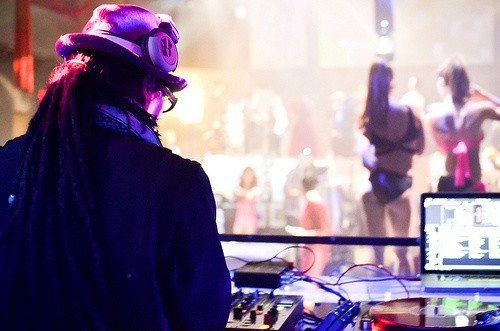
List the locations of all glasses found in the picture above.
[152,76,179,113]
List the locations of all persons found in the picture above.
[351,62,425,277]
[0,4,232,331]
[428,64,500,193]
[283,145,331,236]
[222,166,262,234]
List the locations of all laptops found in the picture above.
[420,192,500,291]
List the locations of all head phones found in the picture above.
[143,14,180,74]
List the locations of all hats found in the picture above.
[52,3,187,93]
